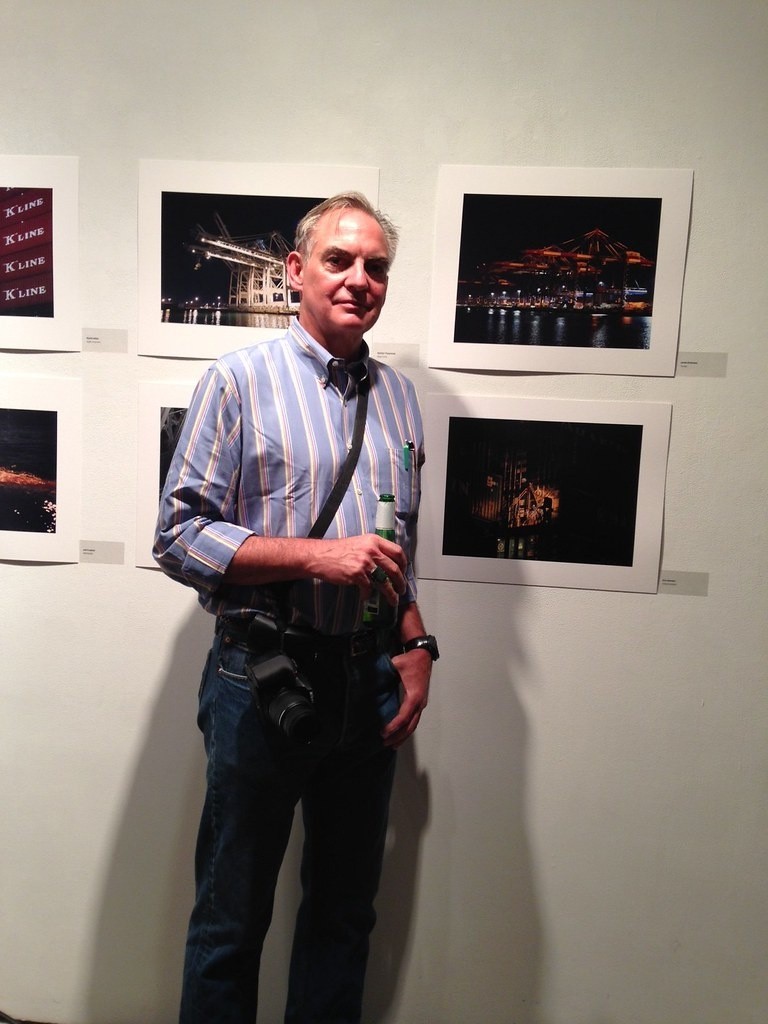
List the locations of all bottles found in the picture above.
[363,495,398,629]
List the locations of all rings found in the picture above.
[369,566,389,584]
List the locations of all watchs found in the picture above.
[402,634,440,662]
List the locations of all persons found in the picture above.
[150,192,432,1024]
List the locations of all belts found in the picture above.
[214,615,396,658]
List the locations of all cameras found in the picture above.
[244,653,320,745]
